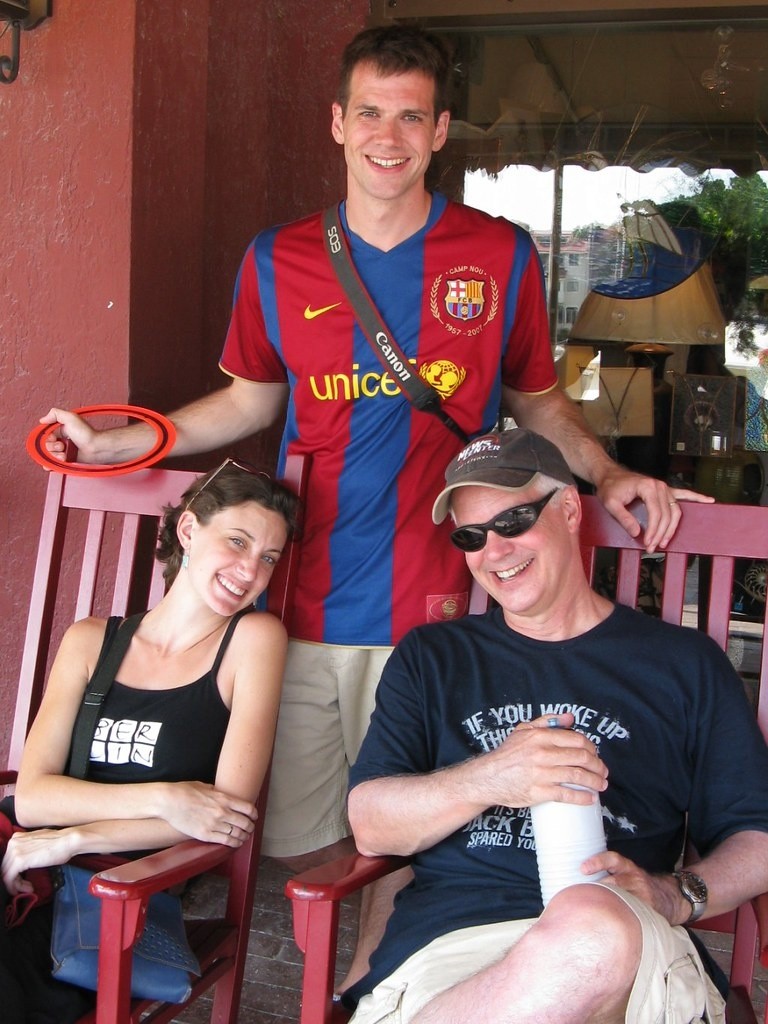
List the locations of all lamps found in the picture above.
[568,261,730,486]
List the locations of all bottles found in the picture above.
[529,716,609,908]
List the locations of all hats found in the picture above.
[432,428,577,525]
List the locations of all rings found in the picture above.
[227,824,233,836]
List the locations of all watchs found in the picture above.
[672,871,707,926]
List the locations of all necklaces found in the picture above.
[599,367,640,462]
[679,376,728,456]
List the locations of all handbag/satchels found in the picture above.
[52,865,202,1004]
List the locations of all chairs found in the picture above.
[285,494,768,1023]
[1,438,311,1024]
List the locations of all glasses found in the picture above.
[449,486,558,553]
[184,458,276,512]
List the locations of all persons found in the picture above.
[340,427,767,1024]
[39,23,716,1001]
[0,457,305,1024]
